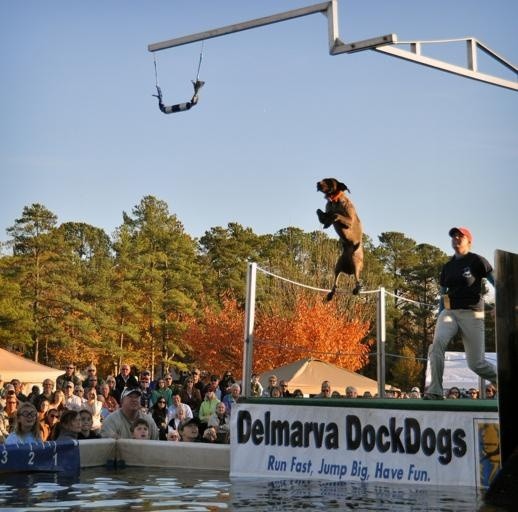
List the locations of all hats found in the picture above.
[4,383,15,394]
[120,386,143,399]
[448,227,473,240]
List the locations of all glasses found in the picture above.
[19,410,37,417]
[65,366,232,404]
[50,414,60,419]
[253,378,494,399]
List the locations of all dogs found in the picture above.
[316,178,364,301]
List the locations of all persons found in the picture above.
[422,227,497,400]
